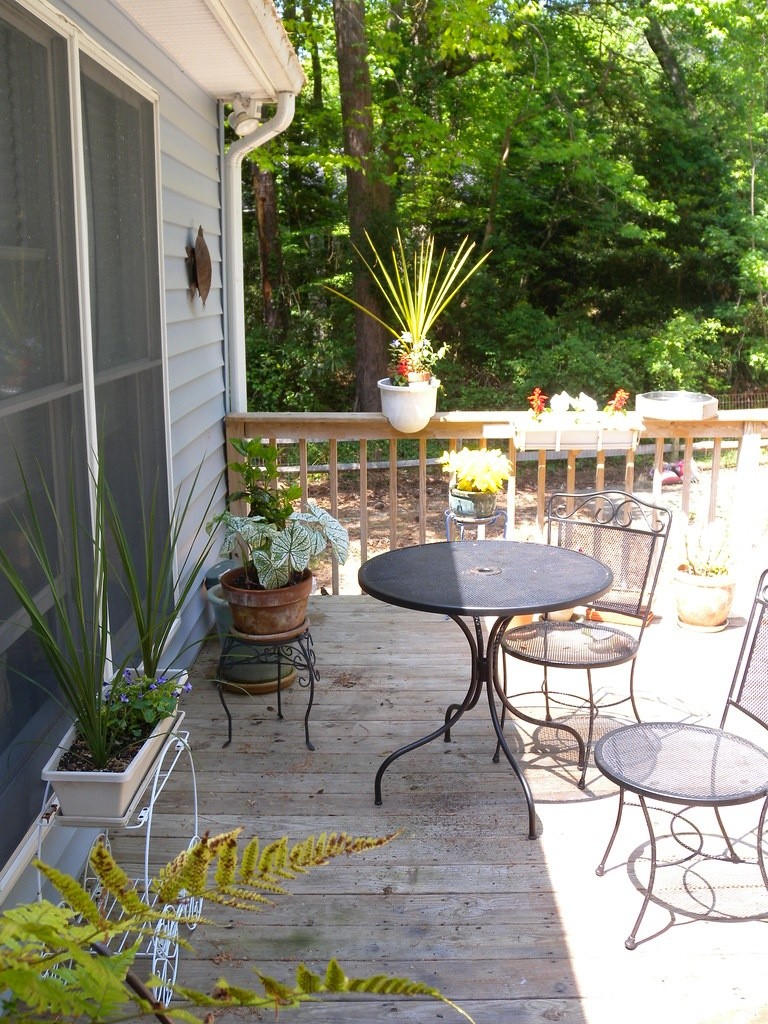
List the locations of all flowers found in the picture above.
[438,446,514,492]
[515,387,648,432]
[101,666,193,739]
[387,331,451,387]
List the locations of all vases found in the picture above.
[448,487,499,517]
[511,416,642,453]
[377,377,441,434]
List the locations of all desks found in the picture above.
[358,540,615,841]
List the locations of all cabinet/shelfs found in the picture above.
[35,731,205,1007]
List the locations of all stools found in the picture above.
[216,616,321,750]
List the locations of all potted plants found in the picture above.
[316,226,495,386]
[672,502,740,626]
[0,402,234,818]
[205,433,302,652]
[205,498,351,632]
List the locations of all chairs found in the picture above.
[491,489,671,790]
[594,570,768,951]
